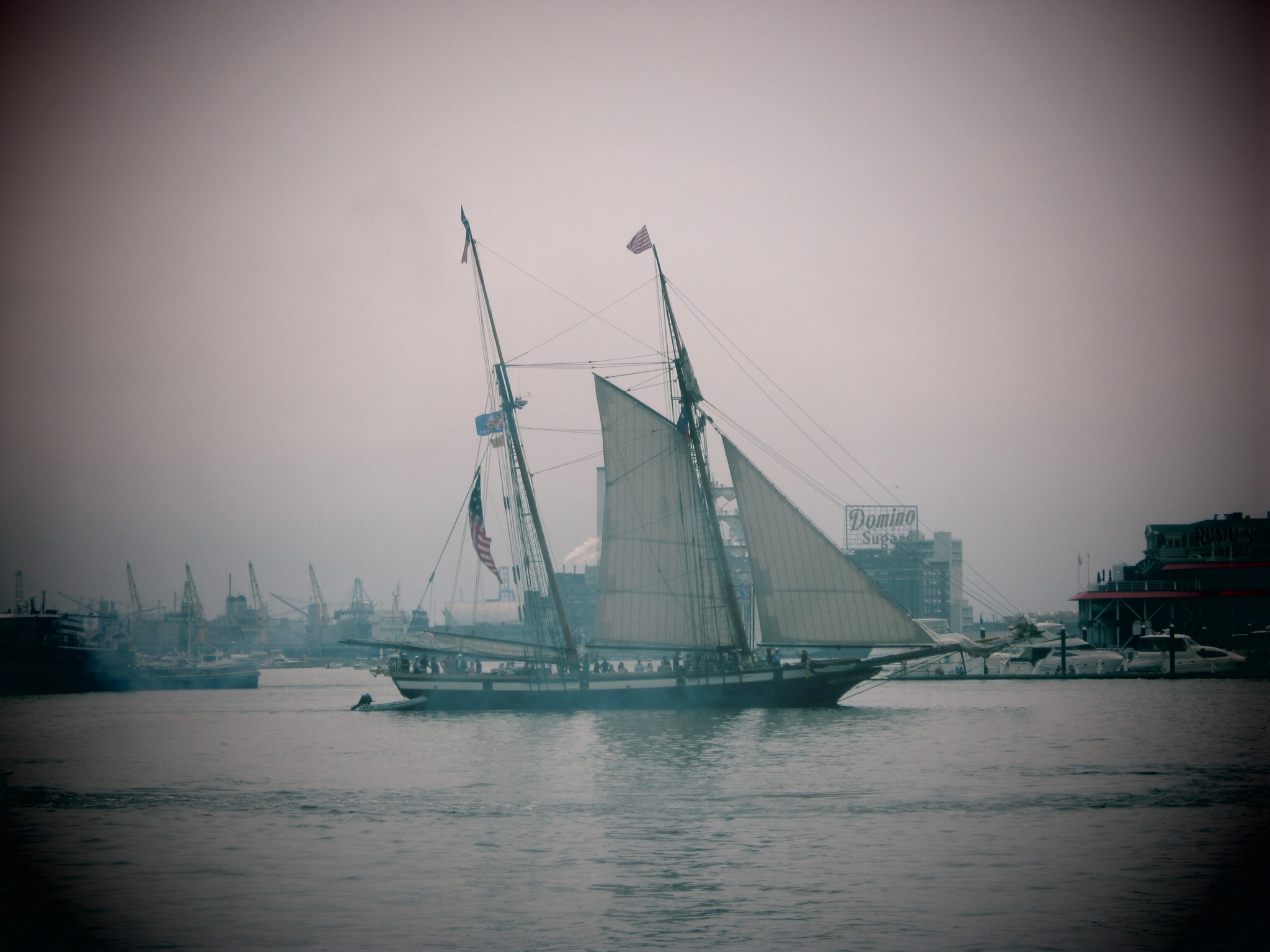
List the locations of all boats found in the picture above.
[260,648,305,668]
[0,573,264,691]
[353,656,372,670]
[359,695,426,712]
[325,660,344,669]
[864,617,1249,680]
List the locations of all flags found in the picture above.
[1079,555,1082,564]
[469,472,501,584]
[461,207,470,263]
[627,226,652,254]
[490,434,505,448]
[475,410,503,436]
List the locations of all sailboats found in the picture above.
[347,201,1040,713]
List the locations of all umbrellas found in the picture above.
[1109,569,1112,581]
[1102,569,1105,581]
[1097,572,1102,584]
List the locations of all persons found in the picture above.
[420,654,431,673]
[440,652,482,674]
[634,659,644,672]
[412,656,422,672]
[497,661,529,675]
[955,666,964,675]
[431,657,440,673]
[400,653,411,673]
[644,662,653,672]
[798,650,808,662]
[579,654,628,673]
[661,651,727,671]
[764,648,782,667]
